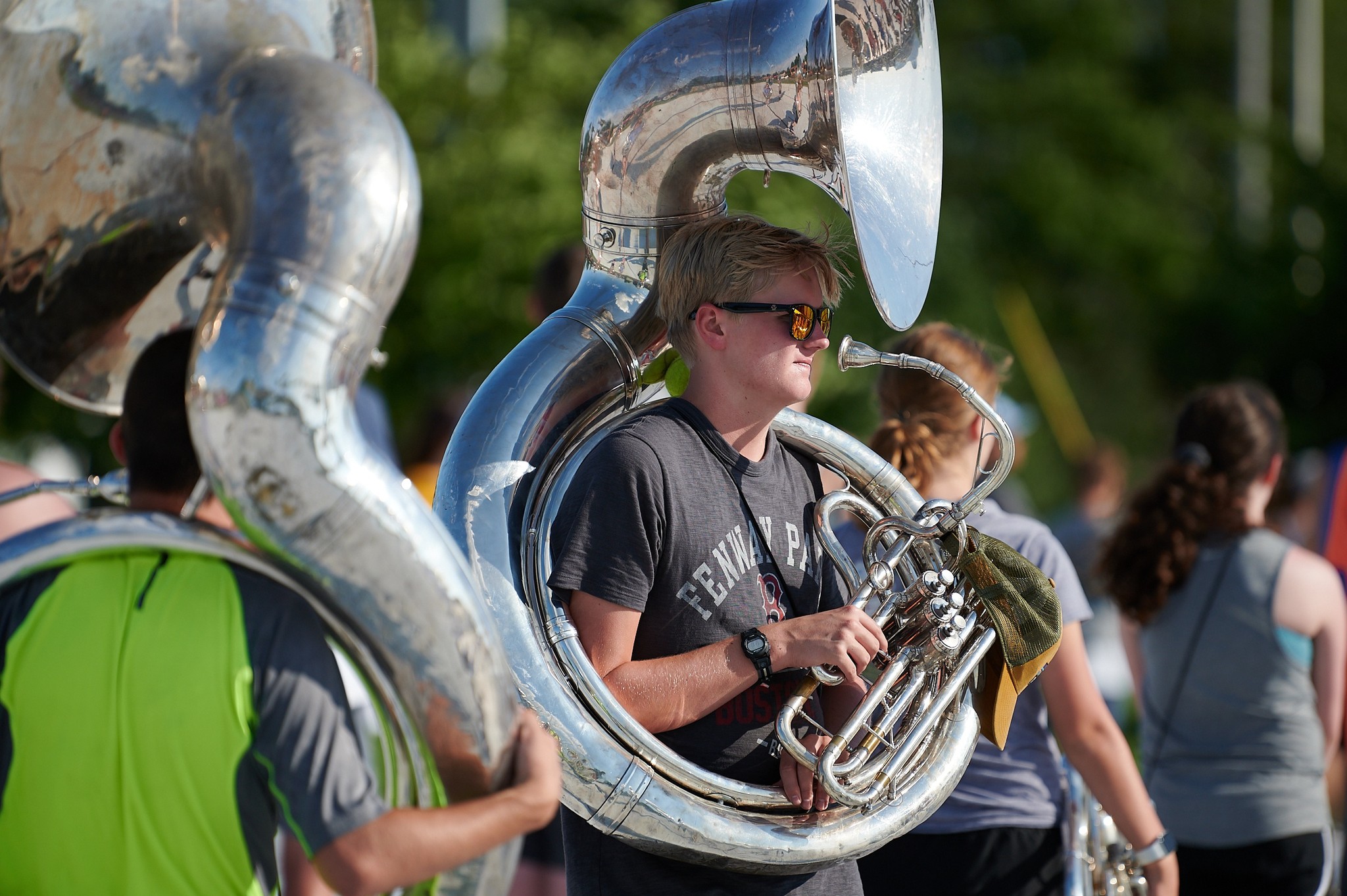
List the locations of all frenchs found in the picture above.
[431,0,1014,876]
[0,0,525,896]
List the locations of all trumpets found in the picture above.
[1061,753,1156,896]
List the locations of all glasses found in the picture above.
[685,300,833,342]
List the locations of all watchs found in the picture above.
[742,627,773,685]
[1136,832,1175,867]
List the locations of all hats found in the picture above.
[944,522,1062,751]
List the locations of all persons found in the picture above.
[973,397,1137,856]
[0,325,564,896]
[1099,378,1347,896]
[555,210,890,896]
[816,321,1183,896]
